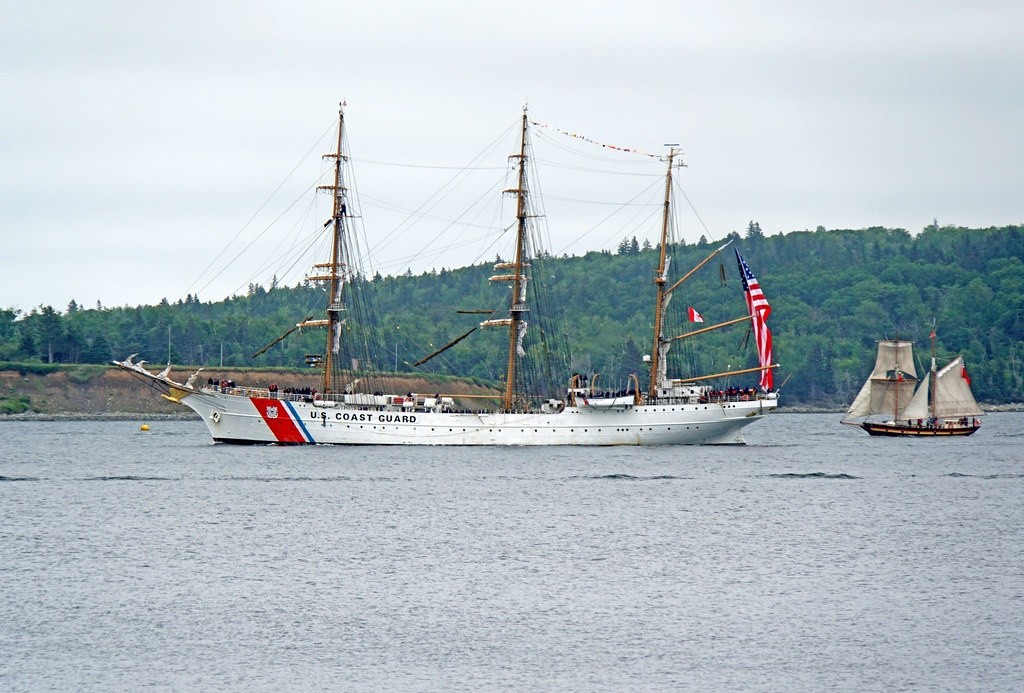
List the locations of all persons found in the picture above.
[926,416,939,429]
[374,390,384,397]
[958,416,969,427]
[767,385,775,394]
[434,392,440,398]
[565,389,643,407]
[918,419,923,428]
[221,379,235,394]
[269,383,278,398]
[972,415,975,427]
[208,377,219,391]
[699,386,758,404]
[282,386,359,401]
[908,419,912,426]
[407,391,414,401]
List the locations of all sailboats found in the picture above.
[112,100,781,449]
[840,315,986,437]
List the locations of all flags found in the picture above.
[688,307,704,323]
[733,243,774,394]
